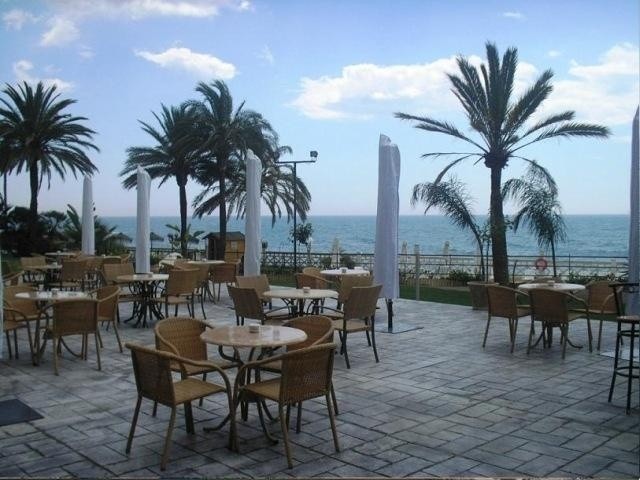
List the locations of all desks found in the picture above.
[200,323,306,449]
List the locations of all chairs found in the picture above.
[479,271,627,359]
[153,317,246,417]
[609,285,639,415]
[122,339,234,471]
[232,341,343,471]
[254,314,339,430]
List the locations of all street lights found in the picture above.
[271,150,319,278]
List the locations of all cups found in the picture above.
[303,287,310,293]
[547,281,555,286]
[248,324,259,333]
[341,269,346,273]
[202,259,206,262]
[51,288,58,295]
[147,272,153,278]
[52,262,57,266]
